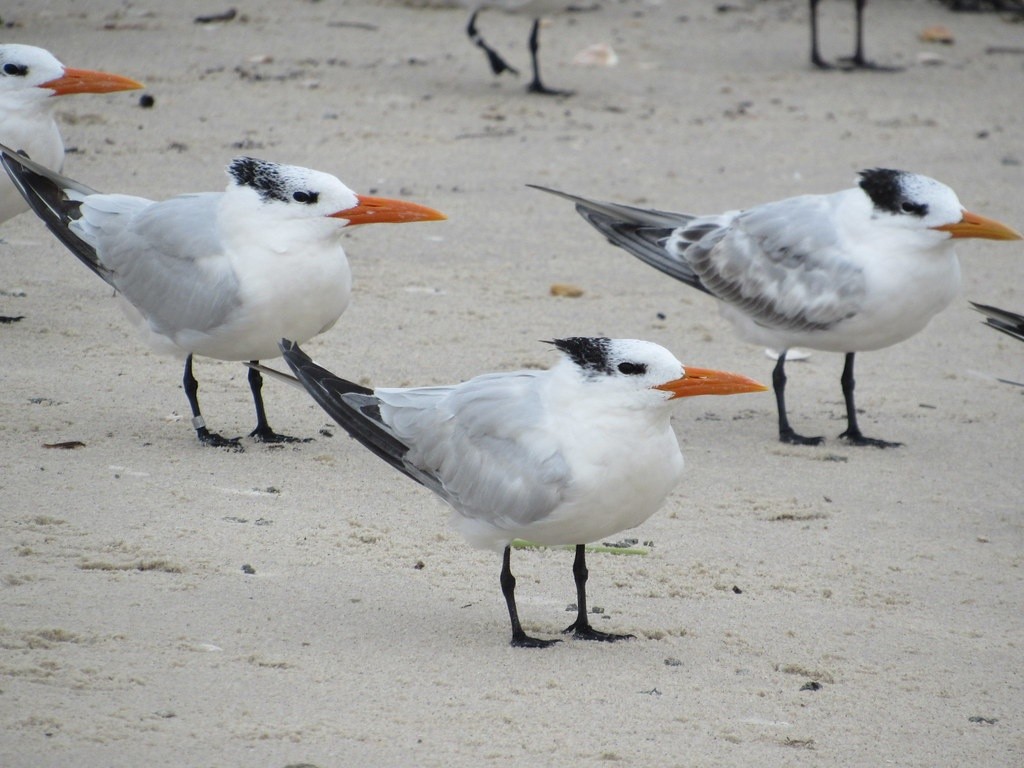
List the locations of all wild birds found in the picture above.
[0,0,1023,653]
